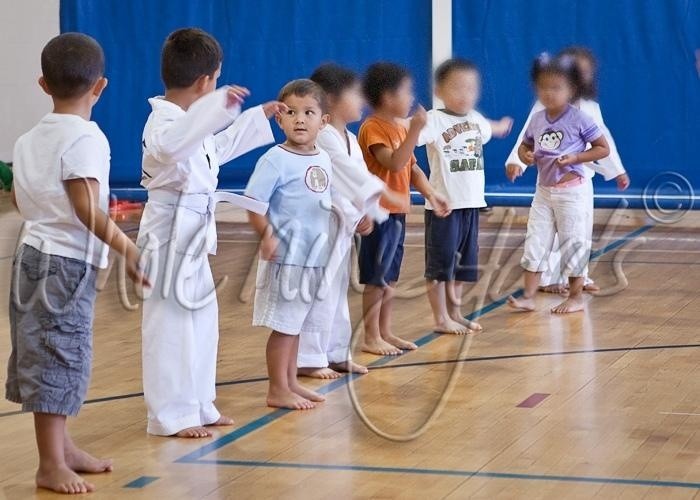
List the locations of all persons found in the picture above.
[2,31,156,495]
[133,25,288,440]
[504,51,611,315]
[294,62,410,381]
[500,45,632,295]
[355,59,455,357]
[243,79,336,411]
[393,57,512,336]
